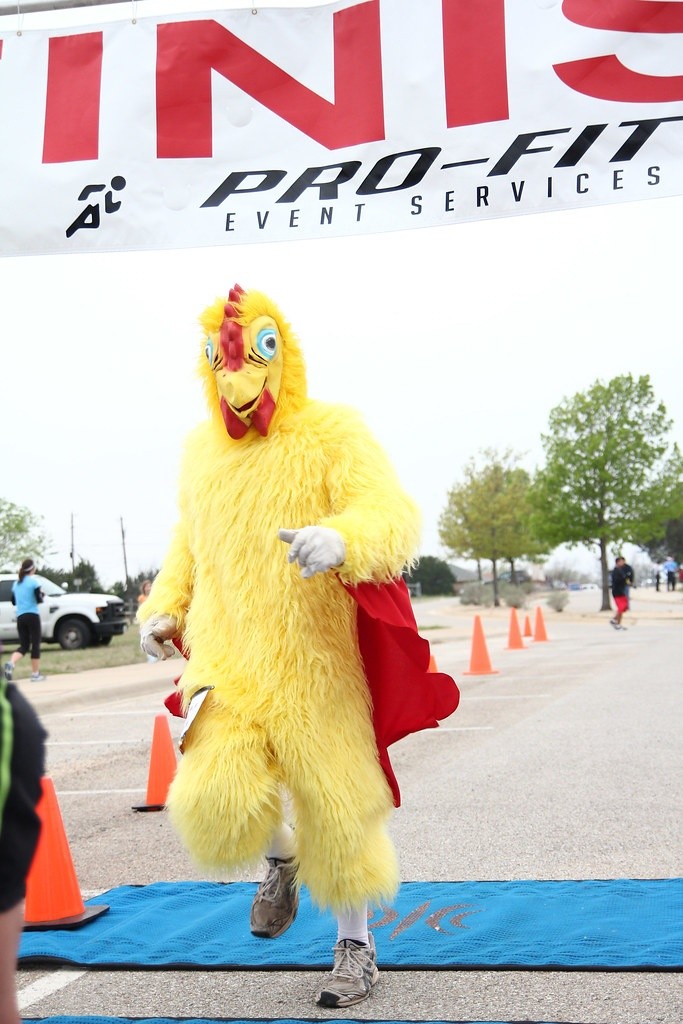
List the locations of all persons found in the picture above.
[136,283,420,1007]
[621,558,634,600]
[138,580,152,612]
[611,558,632,629]
[3,560,44,681]
[661,555,678,591]
[679,560,683,583]
[0,673,48,1024]
[654,560,661,591]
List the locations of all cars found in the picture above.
[551,580,599,591]
[483,570,531,585]
[0,574,130,652]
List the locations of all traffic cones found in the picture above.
[502,606,530,649]
[428,654,439,673]
[531,607,551,642]
[521,616,535,637]
[131,713,178,811]
[462,614,501,676]
[19,774,110,932]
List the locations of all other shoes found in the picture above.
[31,674,44,682]
[611,620,622,630]
[4,662,15,681]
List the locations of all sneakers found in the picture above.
[317,931,379,1009]
[250,856,301,937]
